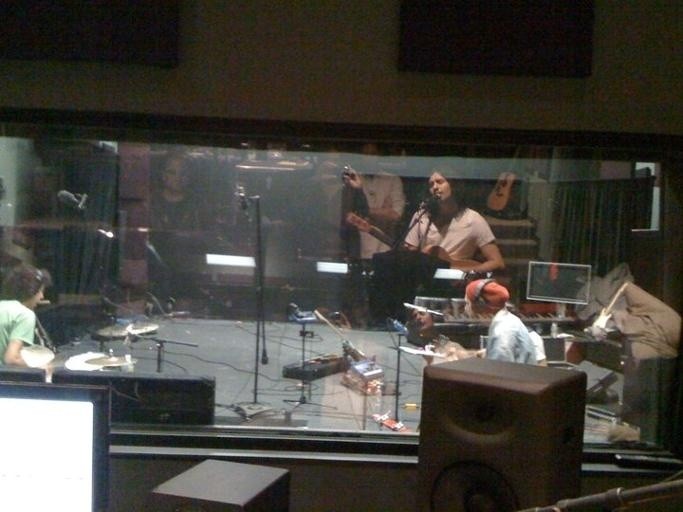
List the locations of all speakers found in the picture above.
[418,359,587,512]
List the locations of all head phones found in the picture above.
[472,278,498,308]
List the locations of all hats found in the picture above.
[467,279,510,308]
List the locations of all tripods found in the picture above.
[283,325,339,415]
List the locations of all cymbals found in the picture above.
[86,356,137,369]
[96,321,160,338]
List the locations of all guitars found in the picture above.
[484,145,523,213]
[343,211,487,297]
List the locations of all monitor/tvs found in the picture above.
[0,380,110,512]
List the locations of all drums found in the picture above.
[64,352,108,372]
[20,344,54,367]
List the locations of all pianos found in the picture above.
[413,296,594,335]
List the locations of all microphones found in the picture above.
[56,189,79,210]
[422,194,441,206]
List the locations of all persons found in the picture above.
[404,308,460,368]
[396,165,507,282]
[25,135,407,329]
[0,261,50,366]
[442,275,537,367]
